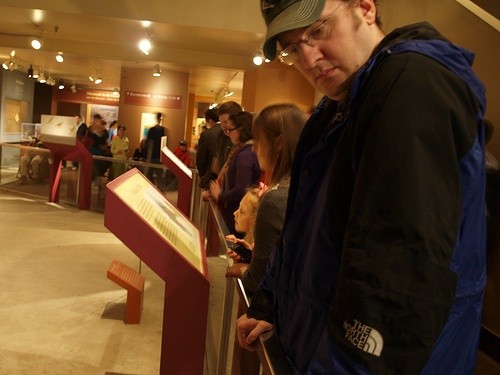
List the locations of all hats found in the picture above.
[260,0,327,60]
[94,114,103,118]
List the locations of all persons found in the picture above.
[73,113,112,176]
[19,134,53,185]
[202,110,261,235]
[480,117,499,270]
[217,100,246,168]
[148,113,168,183]
[108,121,118,142]
[235,0,488,374]
[197,110,227,188]
[173,140,195,169]
[110,124,132,179]
[225,185,269,261]
[133,135,151,163]
[225,103,308,297]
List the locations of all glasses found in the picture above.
[225,125,240,134]
[274,2,343,66]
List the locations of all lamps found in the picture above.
[253,49,263,65]
[3,50,16,70]
[56,50,64,63]
[153,64,161,77]
[70,82,75,89]
[29,65,56,86]
[31,30,46,49]
[113,87,119,95]
[57,79,65,89]
[94,75,102,85]
[88,72,95,82]
[225,85,232,97]
